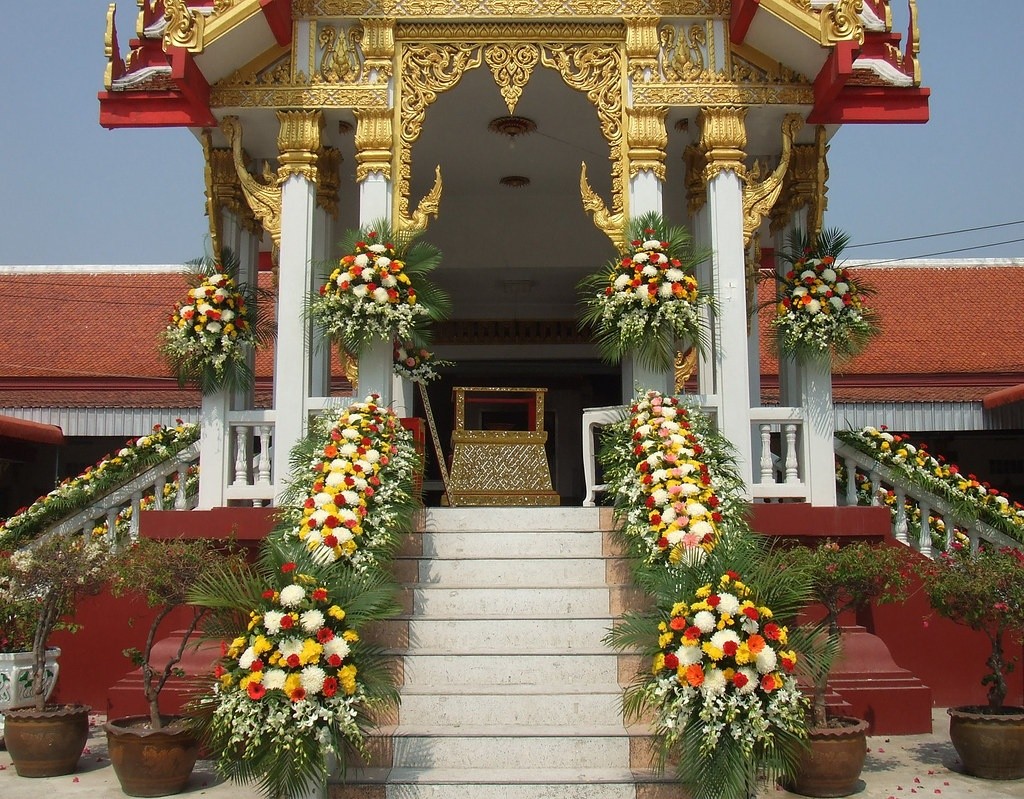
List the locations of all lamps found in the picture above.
[486,117,538,150]
[500,176,530,190]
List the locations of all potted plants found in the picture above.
[0,528,251,799]
[774,536,918,797]
[918,541,1024,781]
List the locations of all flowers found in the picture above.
[0,417,200,557]
[302,217,454,385]
[177,389,426,799]
[834,424,1024,553]
[572,211,726,370]
[591,379,842,799]
[153,230,277,396]
[750,225,885,378]
